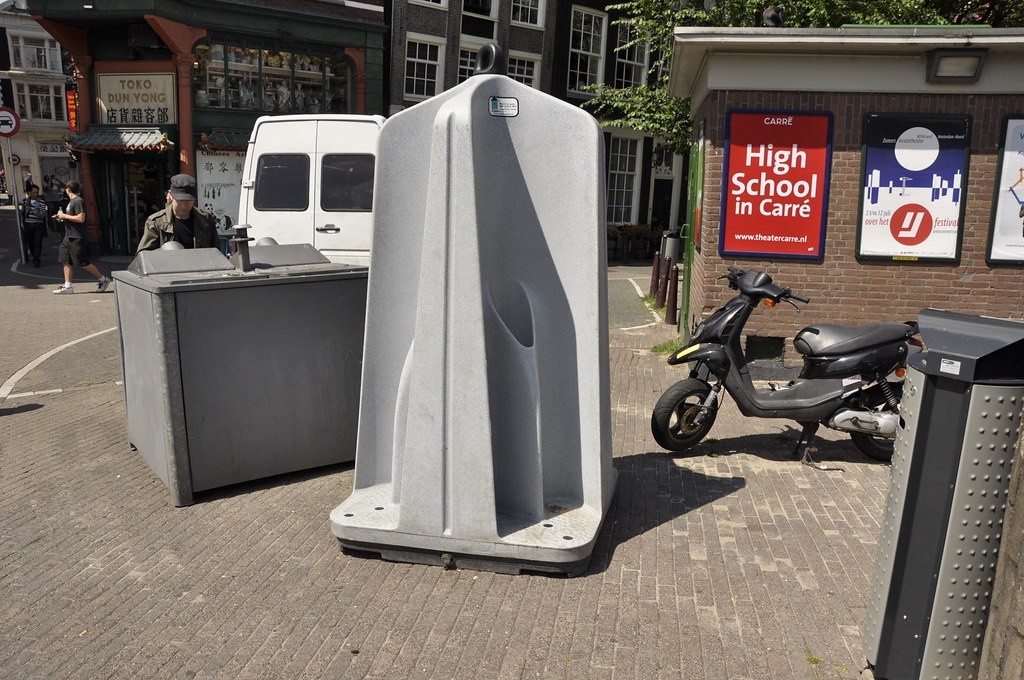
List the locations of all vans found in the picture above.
[238,114,391,265]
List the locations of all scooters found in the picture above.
[651,264,923,460]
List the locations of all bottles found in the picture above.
[57,207,64,223]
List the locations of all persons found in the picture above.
[136,174,221,251]
[23,172,34,199]
[52,181,111,295]
[42,175,65,232]
[22,184,47,268]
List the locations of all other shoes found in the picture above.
[33,260,39,268]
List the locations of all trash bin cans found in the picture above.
[659,227,680,281]
[110,224,368,508]
[865,306,1024,680]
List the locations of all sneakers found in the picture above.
[53,285,74,294]
[97,276,110,292]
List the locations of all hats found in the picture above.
[170,173,197,202]
